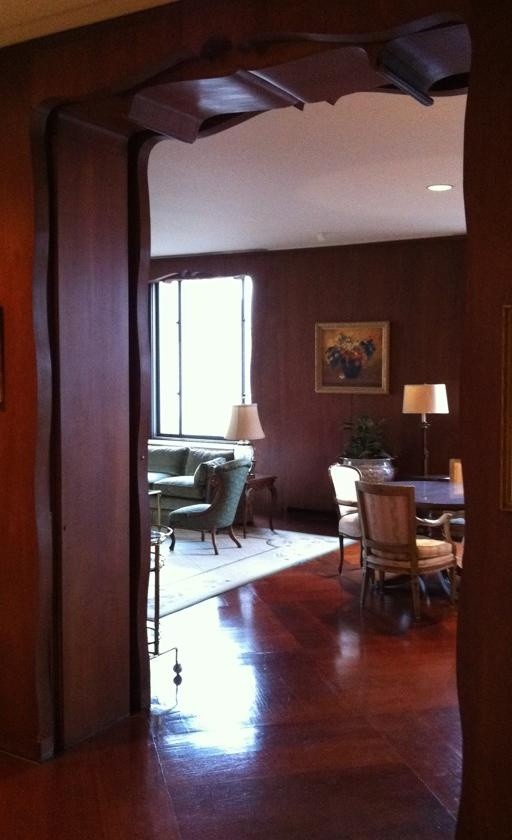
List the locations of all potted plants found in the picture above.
[339,417,397,483]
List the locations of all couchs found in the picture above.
[145,437,237,524]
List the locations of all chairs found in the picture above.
[438,455,468,540]
[353,477,459,621]
[168,458,253,556]
[327,453,367,575]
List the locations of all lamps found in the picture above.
[224,393,265,479]
[400,383,450,476]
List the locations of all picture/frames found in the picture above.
[312,322,392,396]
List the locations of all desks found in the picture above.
[146,489,163,526]
[241,471,278,540]
[385,479,467,599]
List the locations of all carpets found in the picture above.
[145,513,362,626]
